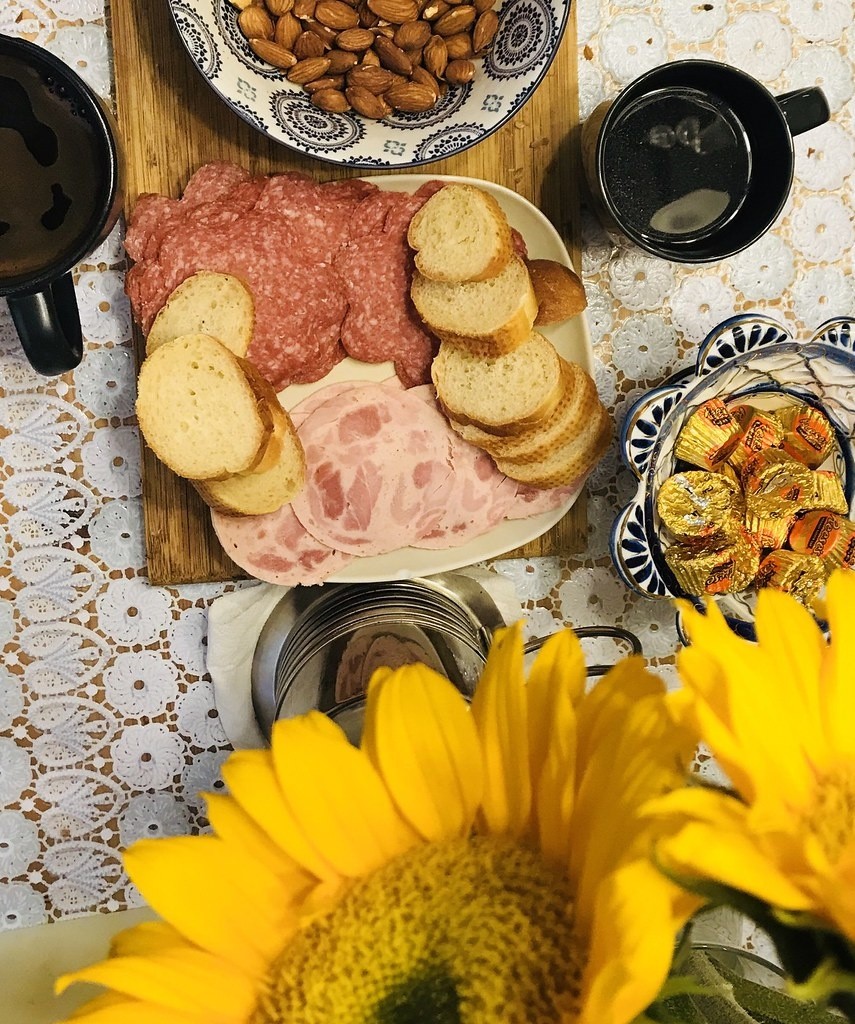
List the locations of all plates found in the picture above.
[168,0,572,170]
[606,312,855,644]
[198,173,594,588]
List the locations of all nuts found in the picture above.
[227,0,500,119]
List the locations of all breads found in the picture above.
[405,182,611,488]
[134,270,309,518]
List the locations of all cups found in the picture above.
[580,57,832,265]
[0,34,128,376]
